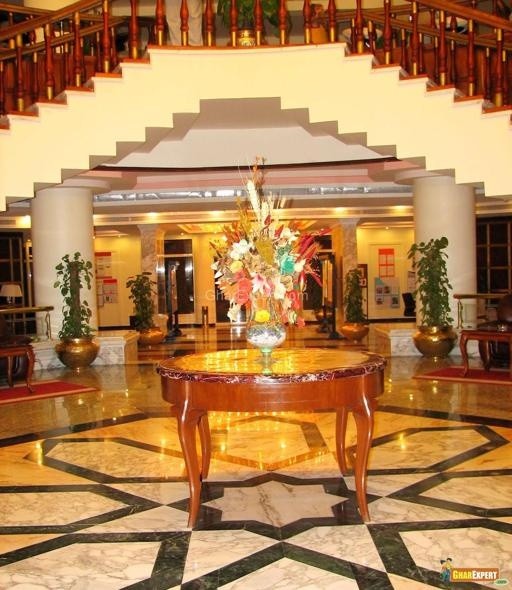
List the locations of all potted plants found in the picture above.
[216,2,290,49]
[341,266,369,343]
[50,252,101,370]
[124,269,165,347]
[409,236,460,362]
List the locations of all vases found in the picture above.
[246,294,286,358]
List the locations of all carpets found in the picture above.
[411,365,512,387]
[1,379,101,404]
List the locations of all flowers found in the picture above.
[205,151,331,331]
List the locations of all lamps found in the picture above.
[0,282,26,336]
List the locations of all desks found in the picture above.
[1,343,39,394]
[457,328,512,375]
[156,346,387,526]
